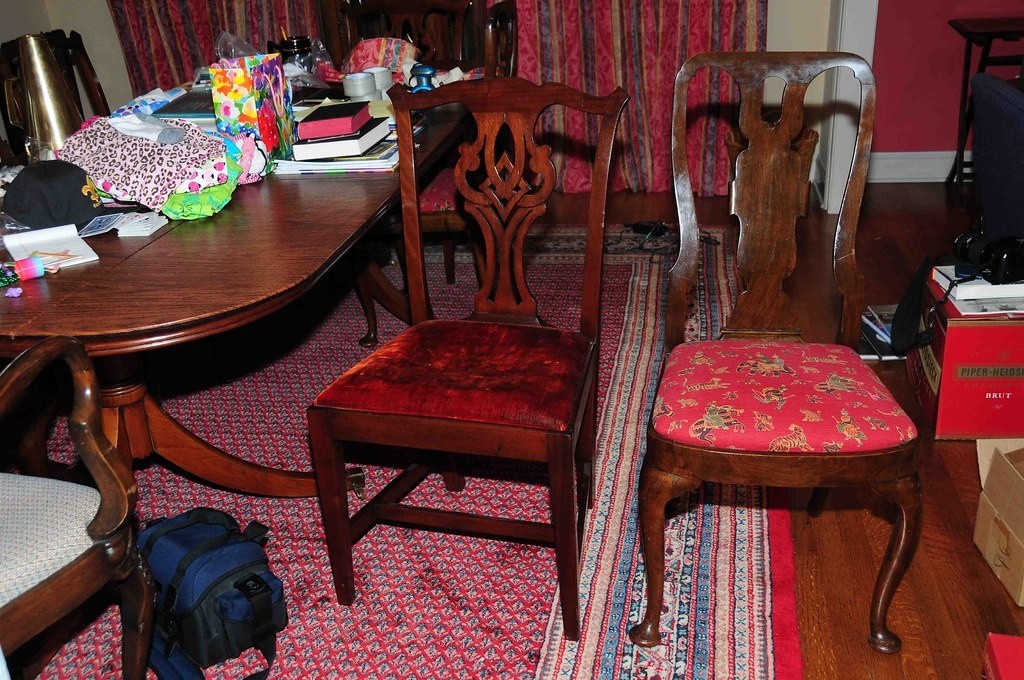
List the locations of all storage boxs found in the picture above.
[906,266,1024,680]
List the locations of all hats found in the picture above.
[2,160,106,229]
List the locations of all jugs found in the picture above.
[5,34,86,164]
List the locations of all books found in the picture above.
[152,90,217,132]
[3,224,99,269]
[271,99,428,174]
[858,305,907,360]
[932,266,1024,315]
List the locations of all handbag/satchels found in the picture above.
[209,52,299,176]
[889,258,933,355]
[130,507,287,680]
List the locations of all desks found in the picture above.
[0,75,472,499]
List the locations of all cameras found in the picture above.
[955,230,1024,284]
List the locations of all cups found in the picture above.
[268,36,312,74]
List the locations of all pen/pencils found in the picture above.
[280,26,287,41]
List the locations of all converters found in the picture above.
[632,221,668,237]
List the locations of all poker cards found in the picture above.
[78,211,169,238]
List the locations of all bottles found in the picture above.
[410,64,436,94]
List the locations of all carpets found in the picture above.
[0,226,800,680]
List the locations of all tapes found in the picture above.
[349,89,383,102]
[380,83,395,101]
[362,66,393,90]
[342,72,376,96]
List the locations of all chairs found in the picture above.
[971,73,1024,248]
[0,0,922,654]
